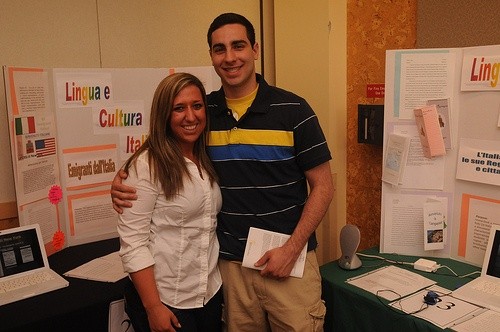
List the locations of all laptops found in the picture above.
[0,224,70,306]
[451,226,500,312]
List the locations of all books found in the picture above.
[63,251,128,282]
[242,227,307,279]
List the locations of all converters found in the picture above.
[415,258,437,272]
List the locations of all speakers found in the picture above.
[338,224,362,270]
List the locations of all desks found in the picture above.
[0,237,132,332]
[319,247,500,332]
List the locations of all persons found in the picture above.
[118,74,221,332]
[111,12,333,332]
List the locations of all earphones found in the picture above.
[424,291,438,305]
